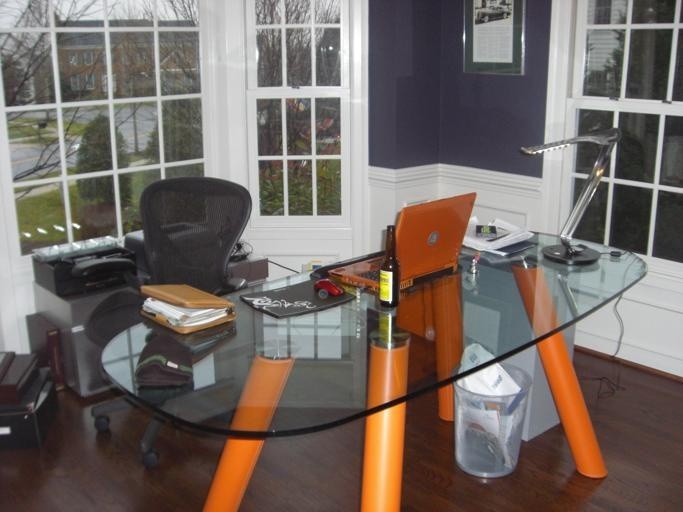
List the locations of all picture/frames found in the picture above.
[461,0,526,76]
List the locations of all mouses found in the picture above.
[314,279,343,299]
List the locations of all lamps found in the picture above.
[521,127,623,266]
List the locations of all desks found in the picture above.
[98,229,648,512]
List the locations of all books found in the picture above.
[137,283,238,335]
[461,215,540,258]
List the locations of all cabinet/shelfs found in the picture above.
[32,286,144,407]
[456,258,579,443]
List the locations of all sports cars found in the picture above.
[475,5,510,23]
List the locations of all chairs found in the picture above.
[83,176,252,471]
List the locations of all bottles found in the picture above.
[377,220,402,308]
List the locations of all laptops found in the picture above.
[329,192,477,295]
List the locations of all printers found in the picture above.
[34,237,136,296]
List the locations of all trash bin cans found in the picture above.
[450,361,533,479]
